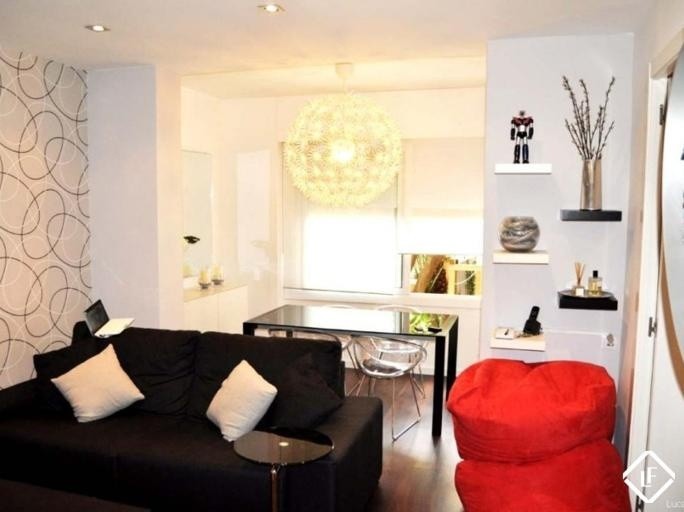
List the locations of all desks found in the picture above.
[240,302,460,442]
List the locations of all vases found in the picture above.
[575,155,608,212]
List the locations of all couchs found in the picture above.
[0,324,385,512]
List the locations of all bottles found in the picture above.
[587,270,602,297]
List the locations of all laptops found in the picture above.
[84,299,136,339]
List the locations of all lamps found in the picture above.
[277,58,409,212]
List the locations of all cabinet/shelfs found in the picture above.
[184,283,246,335]
[489,157,550,352]
[555,204,623,313]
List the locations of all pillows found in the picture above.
[256,350,346,432]
[203,358,281,444]
[48,342,150,424]
[29,337,104,420]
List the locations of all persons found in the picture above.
[510,109,534,164]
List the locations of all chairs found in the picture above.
[267,325,340,350]
[367,304,426,401]
[302,303,361,397]
[353,333,428,444]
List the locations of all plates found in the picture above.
[496,215,541,252]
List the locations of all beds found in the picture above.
[229,424,335,512]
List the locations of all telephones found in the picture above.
[523,305,541,336]
